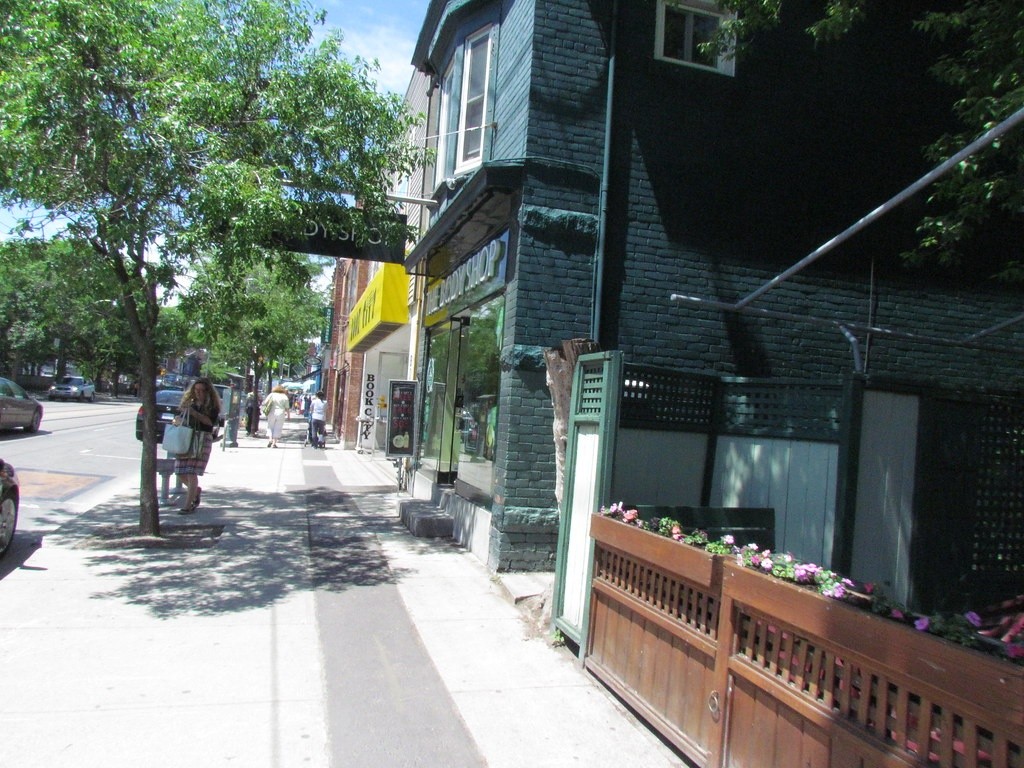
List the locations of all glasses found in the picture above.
[194,388,206,393]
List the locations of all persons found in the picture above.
[245,387,263,435]
[262,385,327,449]
[172,378,221,514]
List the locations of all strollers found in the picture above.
[304,412,326,448]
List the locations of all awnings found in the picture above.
[303,380,316,393]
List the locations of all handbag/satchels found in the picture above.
[162,406,205,460]
[263,393,273,416]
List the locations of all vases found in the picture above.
[754,618,991,768]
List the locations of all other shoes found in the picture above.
[314,445,318,449]
[318,442,322,448]
[268,442,272,448]
[246,432,250,435]
[273,445,278,448]
[255,432,259,435]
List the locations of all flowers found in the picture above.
[600,503,1024,667]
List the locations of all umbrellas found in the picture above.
[282,381,304,390]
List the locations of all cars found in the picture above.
[49,376,95,402]
[136,390,186,443]
[0,376,44,433]
[0,455,22,556]
[211,384,231,426]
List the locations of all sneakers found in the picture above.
[192,487,201,507]
[177,506,195,516]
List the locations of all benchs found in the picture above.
[623,505,777,553]
